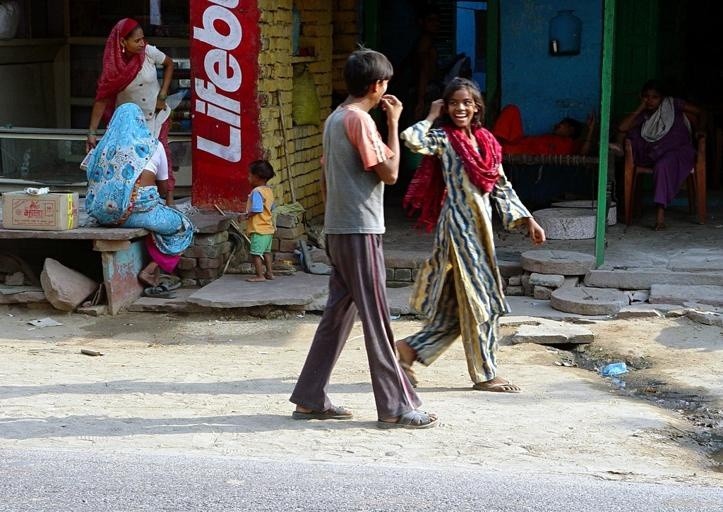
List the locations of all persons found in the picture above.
[615,80,713,232]
[494,102,596,157]
[238,159,276,282]
[81,102,197,290]
[86,18,178,208]
[388,79,551,391]
[282,46,439,429]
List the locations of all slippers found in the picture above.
[394,341,419,388]
[292,404,354,420]
[380,410,441,428]
[473,380,521,393]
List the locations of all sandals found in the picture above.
[139,271,176,298]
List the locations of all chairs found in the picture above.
[625,112,707,226]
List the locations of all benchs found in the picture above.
[0,227,150,316]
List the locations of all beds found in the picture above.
[504,152,617,201]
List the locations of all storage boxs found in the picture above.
[1,189,79,231]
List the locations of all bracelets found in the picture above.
[244,211,249,219]
[86,130,96,137]
[156,95,168,101]
